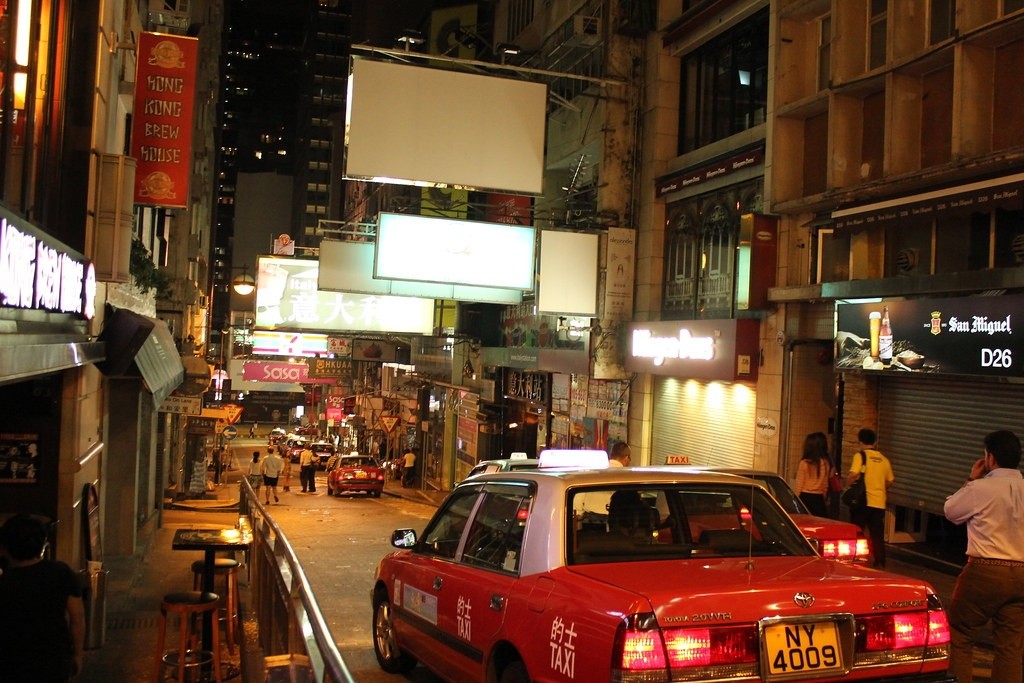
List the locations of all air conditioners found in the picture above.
[563,14,601,47]
[147,0,193,30]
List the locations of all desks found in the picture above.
[173,528,252,683]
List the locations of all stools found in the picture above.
[153,589,222,683]
[191,558,240,656]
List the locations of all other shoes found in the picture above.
[266,501,269,505]
[274,496,279,502]
[308,489,315,492]
[301,489,306,492]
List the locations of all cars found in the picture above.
[269,427,287,444]
[296,421,318,436]
[370,450,950,683]
[310,440,333,469]
[278,433,310,462]
[327,451,384,497]
[641,455,872,569]
[442,452,580,537]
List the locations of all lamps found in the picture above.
[454,28,478,49]
[397,29,425,51]
[497,41,521,63]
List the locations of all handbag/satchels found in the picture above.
[840,450,867,506]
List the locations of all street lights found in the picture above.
[203,265,256,407]
[216,325,228,450]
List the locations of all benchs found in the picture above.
[577,528,751,557]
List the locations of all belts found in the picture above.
[967,557,1024,566]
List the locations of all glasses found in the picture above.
[626,454,631,462]
[606,504,611,511]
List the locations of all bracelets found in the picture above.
[967,477,973,481]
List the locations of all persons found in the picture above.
[605,489,649,538]
[249,452,262,501]
[946,430,1024,683]
[298,443,320,493]
[609,442,631,468]
[397,448,416,487]
[248,426,254,438]
[0,513,86,683]
[261,446,291,504]
[840,428,894,571]
[212,446,226,484]
[793,433,840,519]
